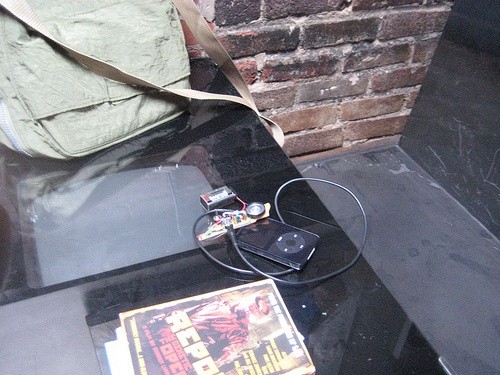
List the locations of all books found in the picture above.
[103,278,317,375]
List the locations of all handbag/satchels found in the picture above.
[0,0,192,159]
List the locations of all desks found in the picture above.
[0,57,451,373]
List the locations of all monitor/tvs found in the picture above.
[16,146,243,291]
